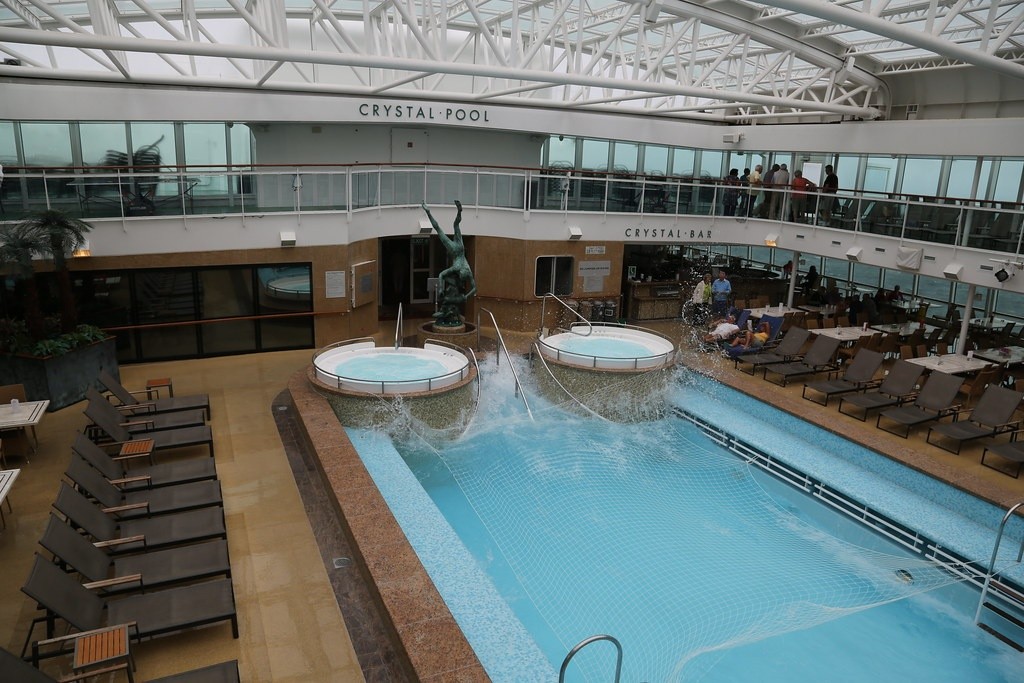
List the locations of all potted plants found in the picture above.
[0,206,122,412]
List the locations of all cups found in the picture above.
[675,274,679,281]
[648,276,652,281]
[766,305,770,313]
[966,351,973,361]
[778,303,783,314]
[641,274,644,279]
[862,322,867,331]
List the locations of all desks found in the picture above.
[593,183,692,215]
[73,624,136,683]
[0,400,50,464]
[0,469,21,529]
[66,178,201,217]
[146,378,173,400]
[75,277,121,309]
[741,286,1024,375]
[119,440,158,470]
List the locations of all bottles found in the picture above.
[836,325,842,335]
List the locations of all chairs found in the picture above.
[689,278,978,439]
[926,322,1024,479]
[805,194,1024,255]
[0,368,242,683]
[125,269,205,331]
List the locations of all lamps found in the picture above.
[845,247,863,262]
[765,234,779,247]
[71,240,91,256]
[568,227,583,240]
[943,264,963,281]
[722,132,742,142]
[995,269,1012,283]
[280,232,296,246]
[418,221,433,233]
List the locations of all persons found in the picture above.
[723,164,789,220]
[819,165,838,226]
[791,171,813,223]
[851,286,904,324]
[421,199,477,303]
[802,266,832,306]
[711,270,732,319]
[728,321,770,350]
[702,315,740,345]
[691,274,714,324]
